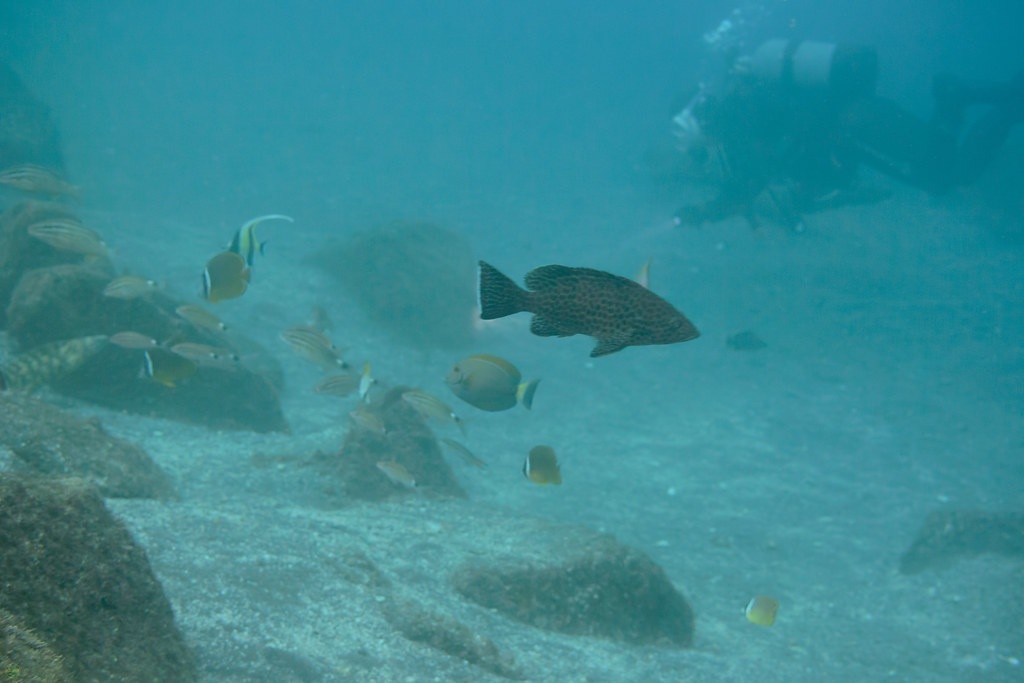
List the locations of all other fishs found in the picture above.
[0,139,778,626]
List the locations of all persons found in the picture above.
[666,62,1023,233]
